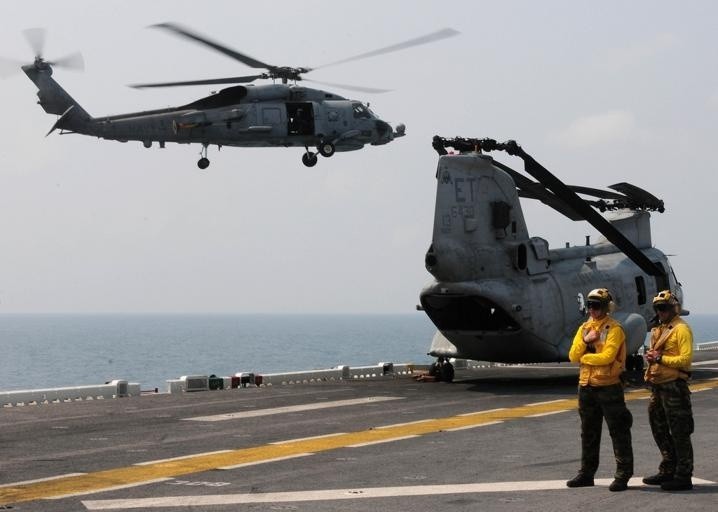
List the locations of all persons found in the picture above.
[641,289,693,491]
[566,287,634,492]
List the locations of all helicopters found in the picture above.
[14,17,461,169]
[419,134,690,386]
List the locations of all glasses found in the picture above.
[652,303,674,312]
[585,300,608,310]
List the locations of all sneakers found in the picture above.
[608,477,630,492]
[642,466,695,492]
[566,472,595,488]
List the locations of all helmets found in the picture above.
[650,290,681,324]
[584,286,616,319]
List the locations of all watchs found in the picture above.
[655,354,662,364]
